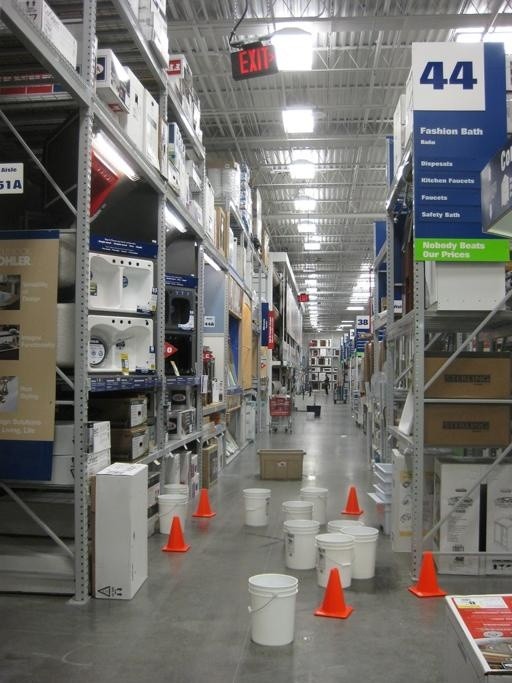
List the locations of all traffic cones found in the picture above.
[408,551,449,599]
[341,484,365,516]
[312,566,353,619]
[161,514,191,553]
[192,488,216,517]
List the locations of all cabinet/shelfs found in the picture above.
[1,0,512,605]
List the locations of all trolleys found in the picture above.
[267,394,293,434]
[331,384,347,404]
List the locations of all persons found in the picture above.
[323,373,329,394]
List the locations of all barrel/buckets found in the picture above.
[340,526,380,580]
[242,488,272,527]
[281,519,320,571]
[280,500,315,520]
[315,532,355,590]
[326,519,365,533]
[300,486,329,526]
[244,573,299,647]
[162,483,189,495]
[156,494,190,536]
